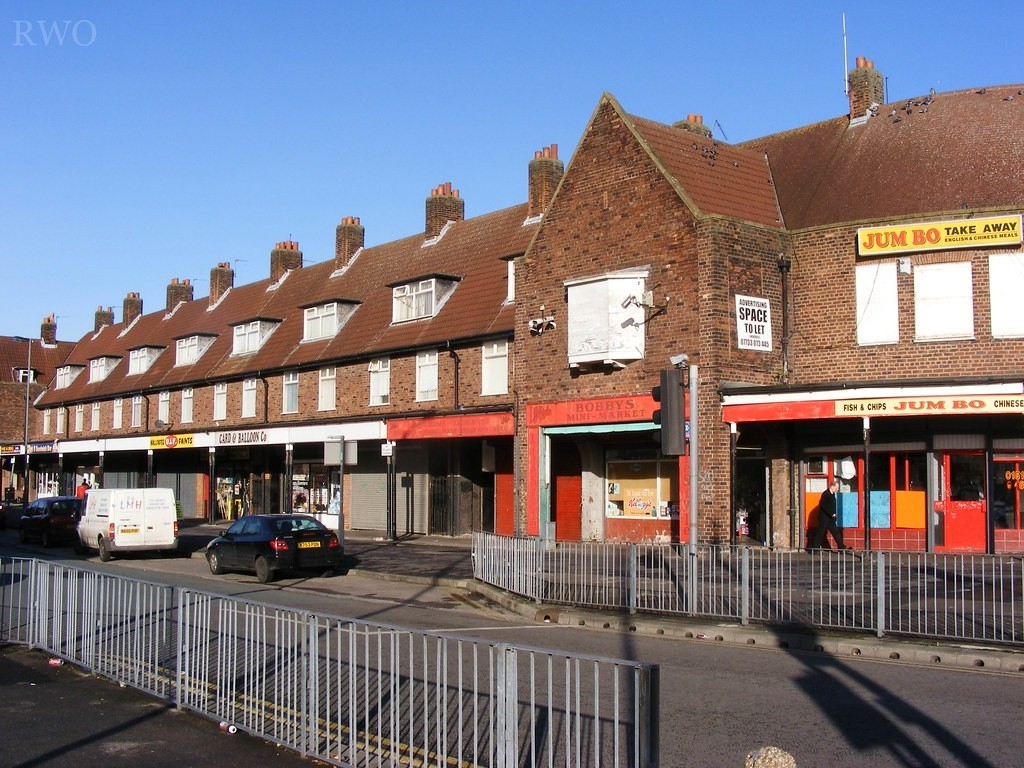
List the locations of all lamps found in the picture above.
[155,420,174,428]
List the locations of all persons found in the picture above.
[82,478,92,490]
[951,468,980,502]
[808,480,854,554]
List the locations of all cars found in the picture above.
[18,495,86,547]
[205,513,340,584]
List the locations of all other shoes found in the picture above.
[805,548,813,554]
[837,547,853,553]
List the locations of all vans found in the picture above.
[72,489,179,561]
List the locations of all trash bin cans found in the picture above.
[4,487,15,501]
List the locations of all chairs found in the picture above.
[279,521,294,532]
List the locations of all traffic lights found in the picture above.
[651,367,687,455]
